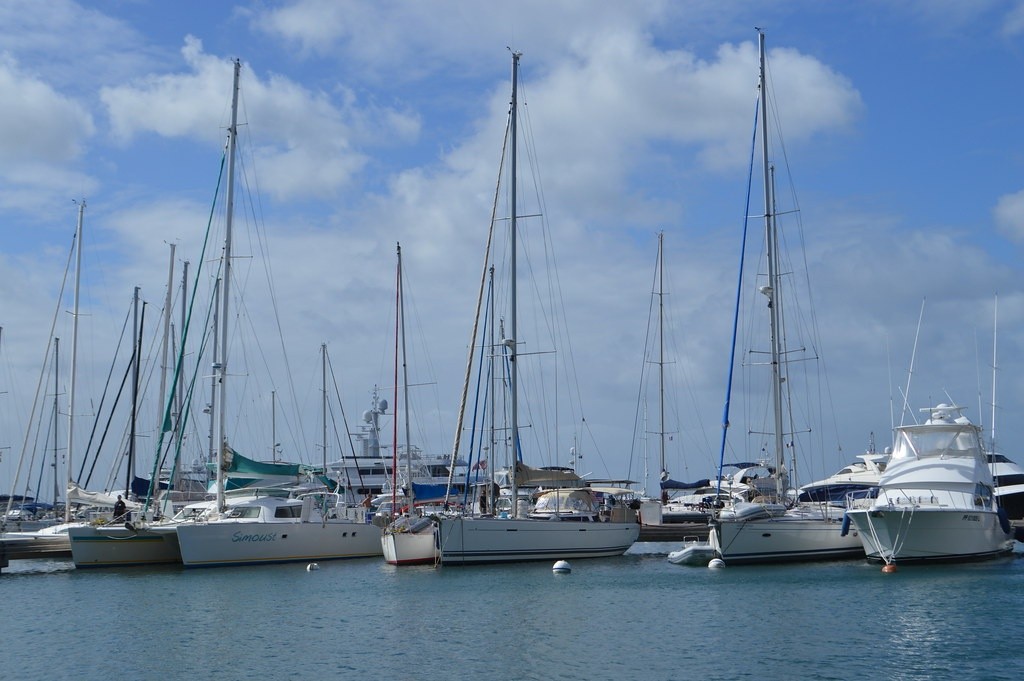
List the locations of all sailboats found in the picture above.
[1,28,1024,570]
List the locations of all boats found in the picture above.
[668,535,714,568]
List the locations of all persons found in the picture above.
[113,494,127,524]
[479,490,486,514]
